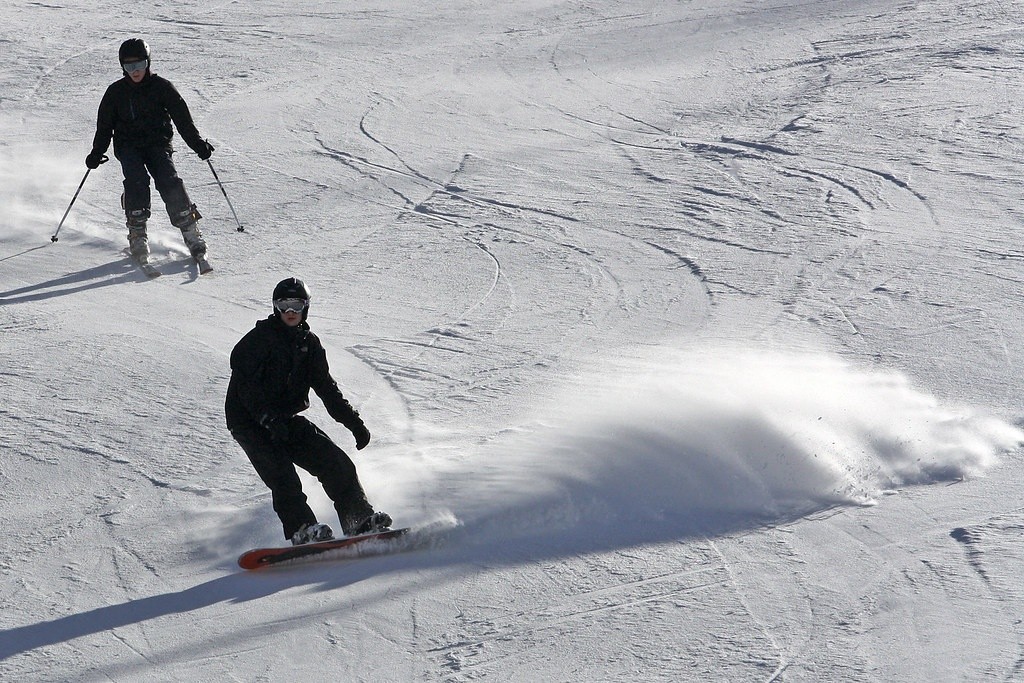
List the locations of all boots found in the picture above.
[126,208,151,256]
[175,207,208,256]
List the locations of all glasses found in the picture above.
[123,59,148,73]
[273,296,312,314]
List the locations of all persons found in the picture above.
[85,38,215,260]
[223,277,393,546]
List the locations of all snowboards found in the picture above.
[237,527,413,570]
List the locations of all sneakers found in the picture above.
[346,511,392,537]
[291,523,333,545]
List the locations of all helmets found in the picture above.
[273,277,311,321]
[119,38,151,76]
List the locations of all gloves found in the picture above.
[345,411,370,449]
[86,149,109,169]
[255,409,289,439]
[196,139,214,160]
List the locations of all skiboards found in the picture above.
[123,241,213,279]
